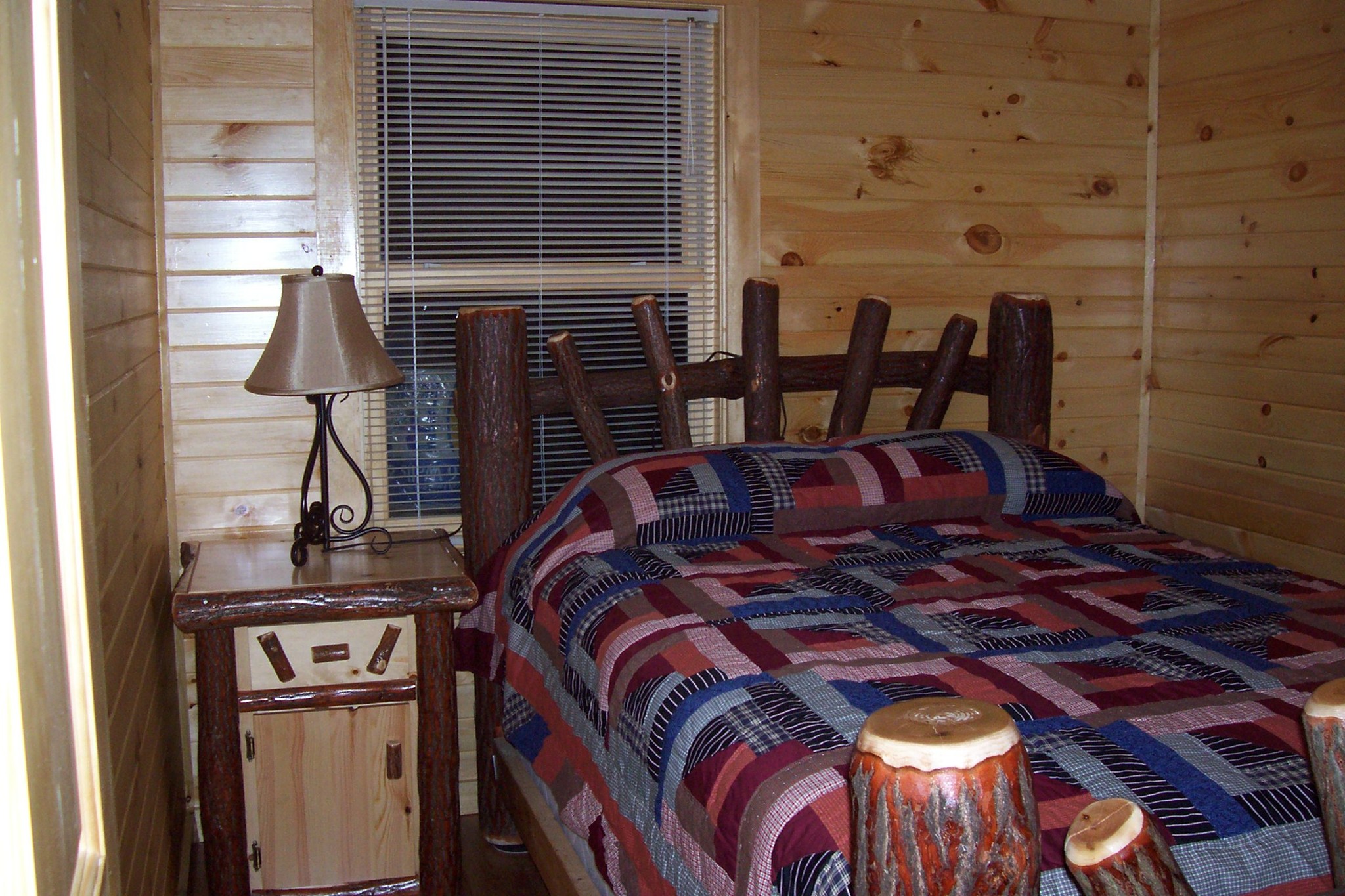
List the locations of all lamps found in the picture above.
[243,264,404,568]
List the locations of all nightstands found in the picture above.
[167,525,480,896]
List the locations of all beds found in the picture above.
[453,273,1345,896]
